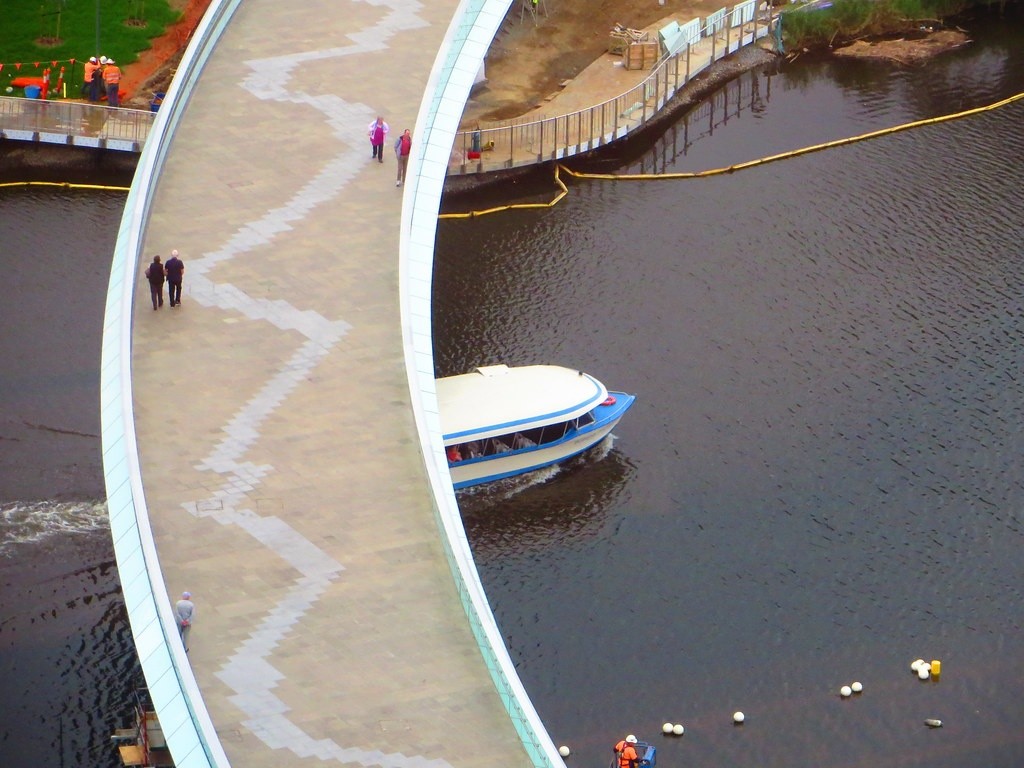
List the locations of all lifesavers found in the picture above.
[601,396,616,405]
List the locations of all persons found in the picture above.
[176,592,193,654]
[81,56,122,109]
[393,128,412,186]
[367,116,389,163]
[448,446,463,462]
[146,256,167,310]
[165,250,184,308]
[613,734,642,768]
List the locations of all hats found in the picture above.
[89,57,97,63]
[107,58,116,64]
[100,56,108,65]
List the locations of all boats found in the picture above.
[432,364,637,491]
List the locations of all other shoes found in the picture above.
[154,306,158,311]
[175,301,181,306]
[171,303,175,309]
[372,155,377,158]
[378,158,383,163]
[396,180,401,186]
[158,300,163,307]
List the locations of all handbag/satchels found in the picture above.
[145,268,152,278]
[367,131,372,137]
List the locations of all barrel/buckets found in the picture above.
[25,86,40,98]
[151,101,160,112]
[157,93,165,100]
